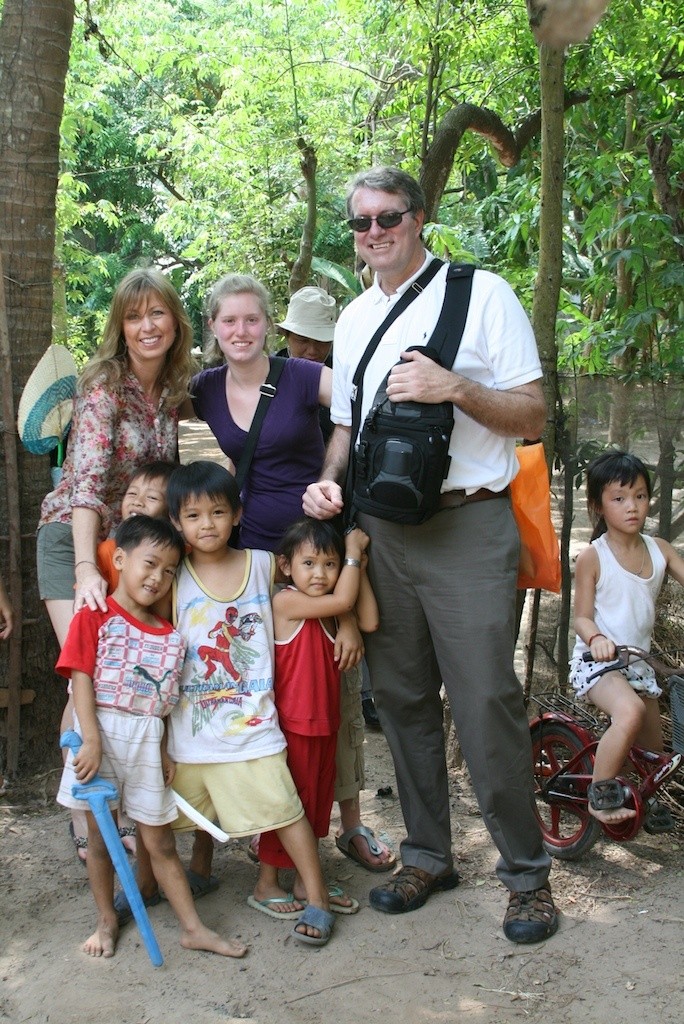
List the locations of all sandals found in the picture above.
[369,859,458,915]
[503,879,558,944]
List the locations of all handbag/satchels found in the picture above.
[510,442,561,594]
[348,262,479,525]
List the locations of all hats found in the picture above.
[273,286,337,342]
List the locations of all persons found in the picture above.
[0,579,13,640]
[568,452,684,825]
[35,165,560,960]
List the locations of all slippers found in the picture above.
[163,870,220,904]
[112,884,159,927]
[291,904,336,945]
[298,886,360,914]
[334,825,397,872]
[246,891,305,920]
[248,833,264,861]
[69,821,89,867]
[116,822,137,855]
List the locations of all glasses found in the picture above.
[347,206,417,233]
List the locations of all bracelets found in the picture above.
[344,558,362,566]
[589,633,608,646]
[74,561,101,576]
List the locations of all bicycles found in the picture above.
[526,644,684,862]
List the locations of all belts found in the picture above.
[436,485,513,509]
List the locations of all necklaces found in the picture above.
[606,536,647,578]
